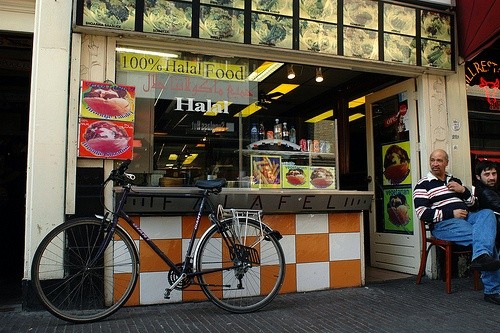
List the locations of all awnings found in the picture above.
[456,0,500,65]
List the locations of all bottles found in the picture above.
[251,119,296,144]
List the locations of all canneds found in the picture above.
[299,139,307,151]
[313,139,330,153]
[267,130,273,139]
[306,140,313,152]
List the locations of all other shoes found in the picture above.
[471,253,500,273]
[484,293,500,305]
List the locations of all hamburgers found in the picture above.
[286,168,306,184]
[84,121,128,154]
[84,86,130,116]
[384,145,410,183]
[387,193,408,225]
[311,168,333,188]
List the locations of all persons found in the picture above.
[413,149,500,305]
[473,159,500,238]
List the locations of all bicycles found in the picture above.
[31,159,286,323]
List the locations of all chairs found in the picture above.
[416,213,479,294]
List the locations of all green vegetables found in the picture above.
[424,16,444,63]
[86,0,325,47]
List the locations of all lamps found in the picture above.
[287,64,295,79]
[315,67,323,82]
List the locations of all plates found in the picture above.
[424,45,447,67]
[90,9,130,26]
[387,10,412,33]
[424,16,447,37]
[386,37,412,61]
[150,10,187,32]
[304,0,332,20]
[347,3,375,26]
[303,29,332,52]
[256,0,288,12]
[257,23,287,44]
[204,18,240,38]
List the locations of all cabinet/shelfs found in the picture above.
[239,110,339,190]
